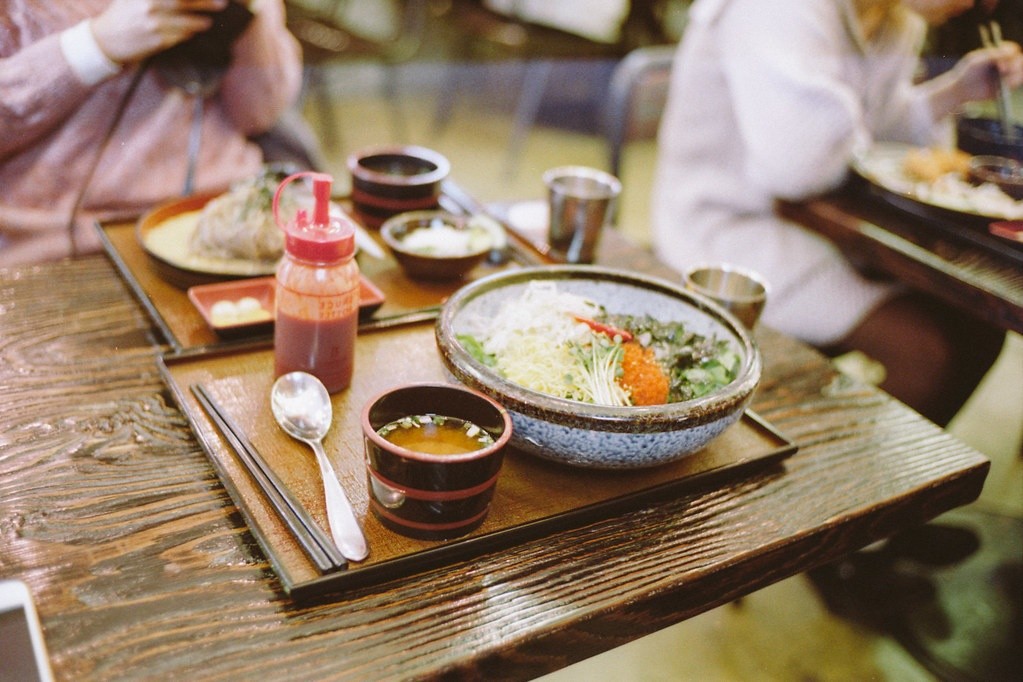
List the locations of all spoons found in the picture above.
[270,371,369,562]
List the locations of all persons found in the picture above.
[0,0,303,267]
[651,0,1023,429]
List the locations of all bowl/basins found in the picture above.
[135,186,335,289]
[967,155,1023,200]
[347,144,450,229]
[435,265,762,470]
[956,117,1023,161]
[360,382,513,542]
[380,209,504,280]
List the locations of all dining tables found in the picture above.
[0,199,1023,682]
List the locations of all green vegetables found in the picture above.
[454,321,739,409]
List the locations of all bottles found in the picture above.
[273,171,359,396]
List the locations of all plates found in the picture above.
[187,276,387,341]
[849,140,1023,221]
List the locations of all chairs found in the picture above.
[607,43,674,219]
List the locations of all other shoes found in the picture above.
[807,520,981,636]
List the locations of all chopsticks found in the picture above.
[979,20,1016,145]
[189,382,348,576]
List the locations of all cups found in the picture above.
[683,268,769,331]
[542,165,622,266]
